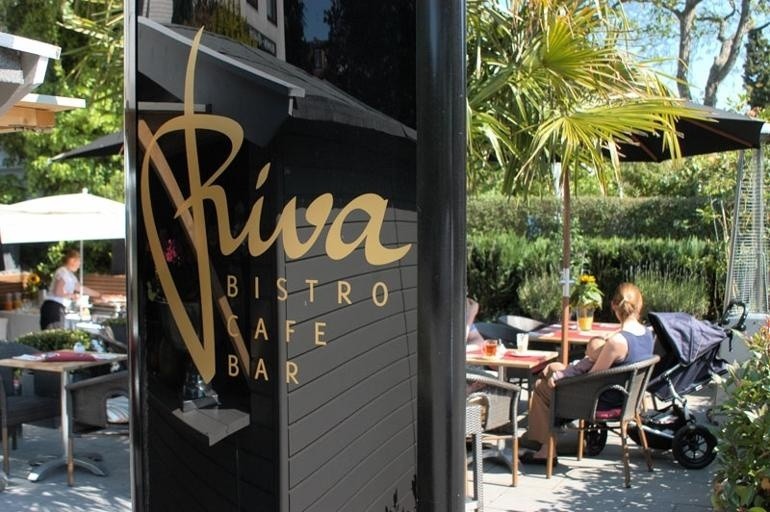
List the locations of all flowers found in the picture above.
[568,274,605,318]
[28,272,41,293]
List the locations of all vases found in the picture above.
[576,306,595,331]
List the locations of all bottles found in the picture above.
[38,282,47,303]
[13,374,20,395]
[4,292,22,311]
[181,372,206,399]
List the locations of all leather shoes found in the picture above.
[519,450,558,466]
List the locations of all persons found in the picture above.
[519,283,655,466]
[547,338,606,389]
[40,247,109,329]
[465,298,488,391]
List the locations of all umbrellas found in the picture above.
[0,187,126,310]
[529,90,765,367]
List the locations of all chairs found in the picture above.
[465,315,660,512]
[0,325,137,486]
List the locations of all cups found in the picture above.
[482,339,498,357]
[516,333,530,352]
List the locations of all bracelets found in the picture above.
[99,293,103,299]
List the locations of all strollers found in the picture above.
[615,300,748,468]
